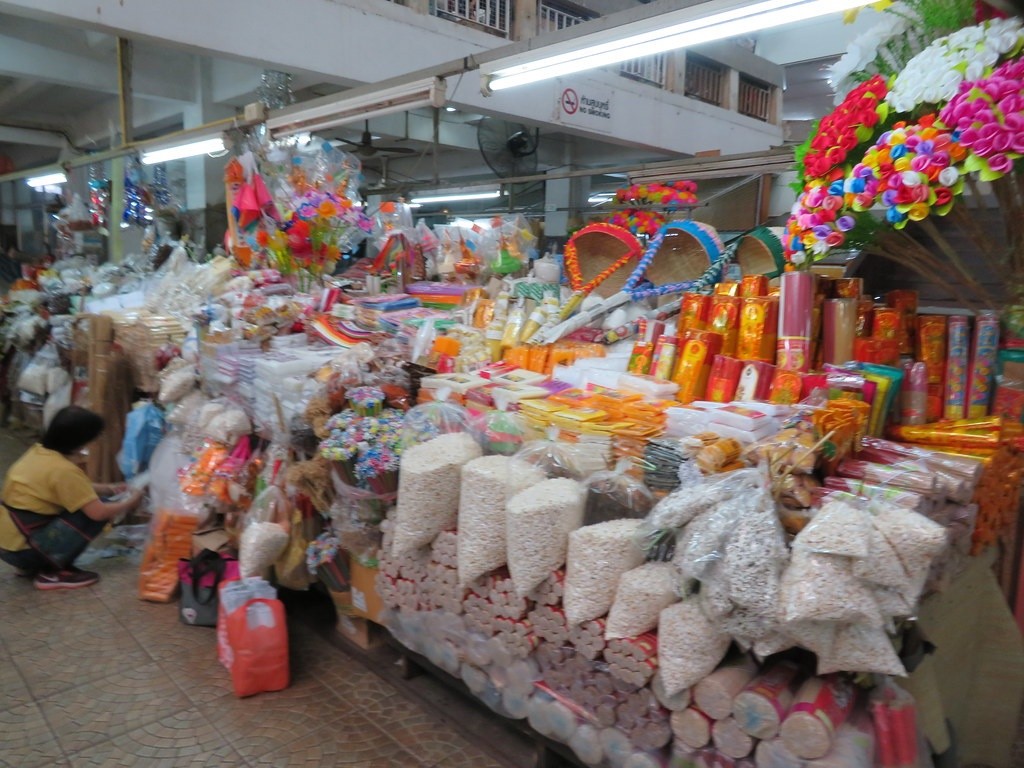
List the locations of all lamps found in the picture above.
[409,183,509,203]
[138,0,883,166]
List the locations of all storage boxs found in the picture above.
[347,558,382,623]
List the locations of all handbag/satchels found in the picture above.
[227,598,289,699]
[177,548,241,627]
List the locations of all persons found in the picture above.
[1,240,23,286]
[152,219,194,274]
[1,404,144,591]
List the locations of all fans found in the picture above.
[335,119,416,156]
[477,114,539,185]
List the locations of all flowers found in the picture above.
[781,0,1024,310]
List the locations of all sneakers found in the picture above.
[34,566,99,589]
[0,547,33,578]
